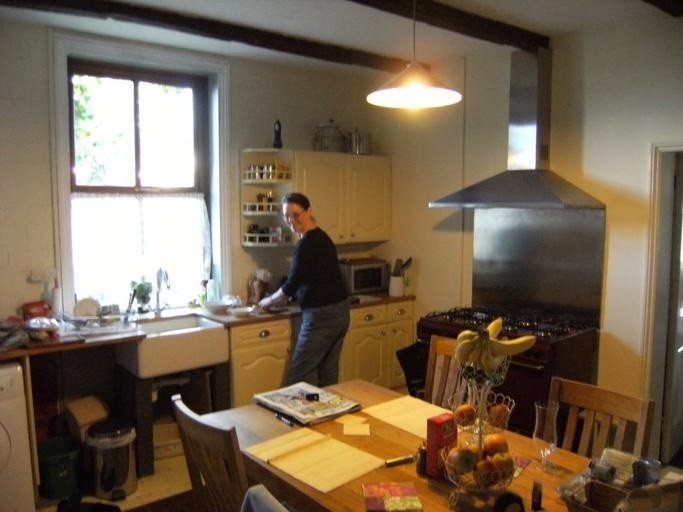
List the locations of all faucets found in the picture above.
[147,267,171,321]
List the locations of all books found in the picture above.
[255,382,361,425]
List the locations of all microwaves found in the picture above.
[339,259,390,296]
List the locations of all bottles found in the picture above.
[267,191,272,210]
[249,161,253,180]
[244,164,247,181]
[416,442,428,476]
[207,279,219,301]
[254,160,259,179]
[52,278,62,315]
[262,164,267,180]
[268,164,272,179]
[274,119,282,148]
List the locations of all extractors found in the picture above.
[428,50,607,214]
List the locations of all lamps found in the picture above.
[365,1,464,113]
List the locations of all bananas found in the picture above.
[455,317,537,370]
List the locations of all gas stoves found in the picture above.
[416,305,596,364]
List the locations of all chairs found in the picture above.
[543,379,653,458]
[420,333,488,411]
[170,392,249,512]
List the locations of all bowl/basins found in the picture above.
[204,301,232,315]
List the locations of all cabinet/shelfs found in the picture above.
[238,144,395,249]
[197,289,420,406]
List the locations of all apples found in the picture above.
[474,460,500,483]
[447,447,476,474]
[457,432,473,449]
[492,452,514,478]
[467,444,481,460]
[482,433,509,458]
[455,404,476,427]
[490,404,510,426]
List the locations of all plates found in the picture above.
[73,298,100,319]
[228,307,253,317]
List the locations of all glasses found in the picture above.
[282,208,308,220]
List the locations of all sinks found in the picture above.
[131,314,228,378]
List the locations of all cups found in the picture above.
[532,399,559,473]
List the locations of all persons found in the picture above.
[258,194,350,388]
